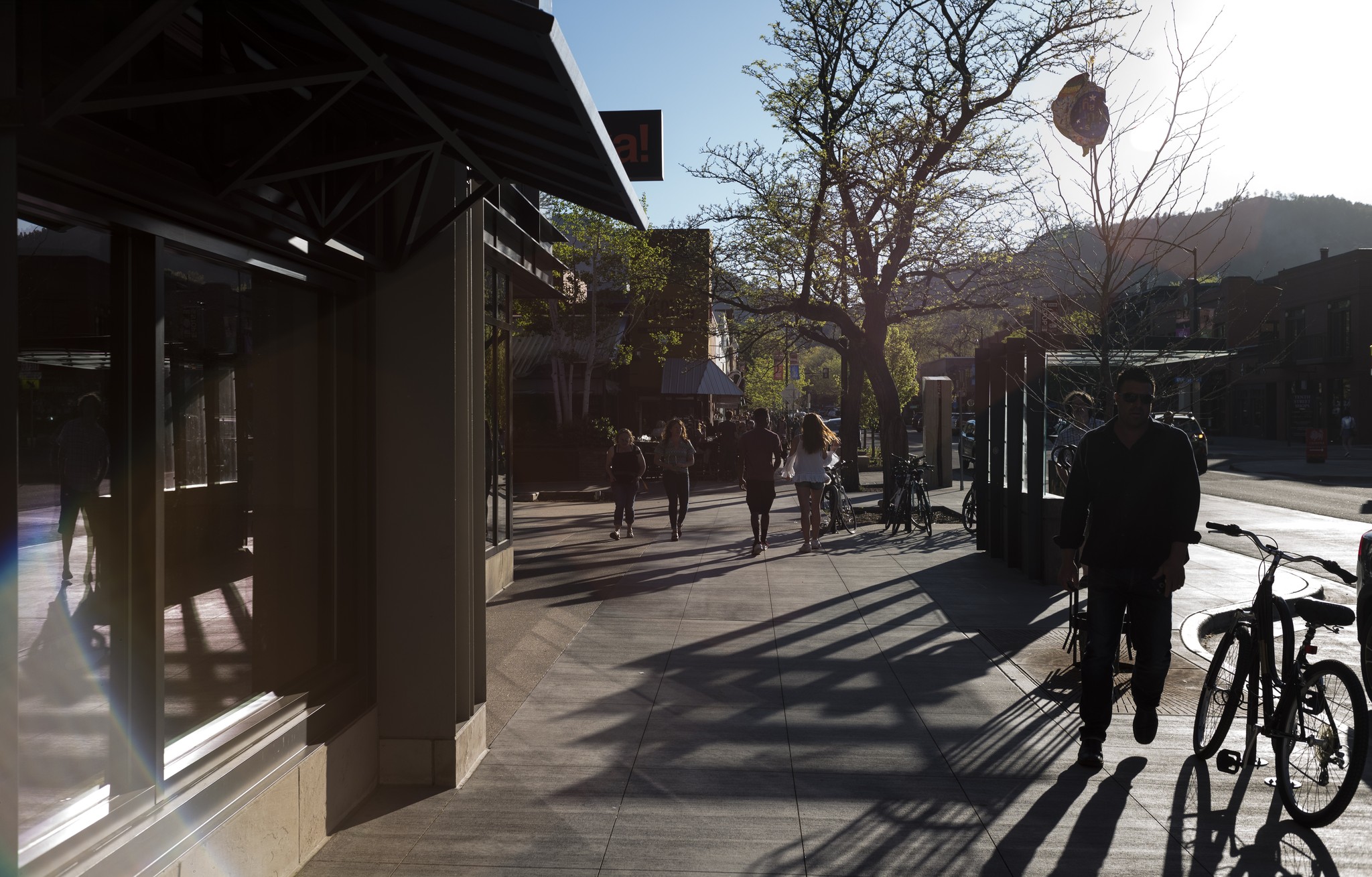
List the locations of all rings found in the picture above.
[1178,585,1181,588]
[1066,578,1069,580]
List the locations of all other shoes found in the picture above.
[732,477,739,482]
[717,478,722,482]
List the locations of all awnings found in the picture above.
[319,0,648,233]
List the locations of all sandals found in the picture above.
[610,531,619,540]
[626,531,635,538]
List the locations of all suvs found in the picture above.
[958,418,975,470]
[912,412,923,432]
[1150,412,1208,474]
[951,413,975,436]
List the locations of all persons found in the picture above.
[654,419,696,541]
[606,428,646,540]
[1052,391,1105,590]
[651,410,802,479]
[1340,412,1356,458]
[738,407,781,555]
[781,414,840,552]
[1058,366,1201,770]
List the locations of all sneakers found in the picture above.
[750,541,763,554]
[1132,704,1158,744]
[799,544,811,552]
[671,531,679,541]
[677,523,682,538]
[762,540,768,550]
[1077,739,1104,768]
[813,539,821,549]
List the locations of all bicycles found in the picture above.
[1192,521,1372,828]
[809,460,857,535]
[879,453,934,538]
[961,472,977,533]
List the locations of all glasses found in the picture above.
[1119,392,1154,404]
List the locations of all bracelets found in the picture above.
[738,476,744,482]
[638,475,641,478]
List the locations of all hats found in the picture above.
[781,434,786,437]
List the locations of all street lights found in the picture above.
[1110,234,1201,338]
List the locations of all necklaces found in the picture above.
[622,449,625,453]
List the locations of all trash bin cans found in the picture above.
[632,441,664,481]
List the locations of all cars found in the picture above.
[823,418,862,450]
[812,408,841,419]
